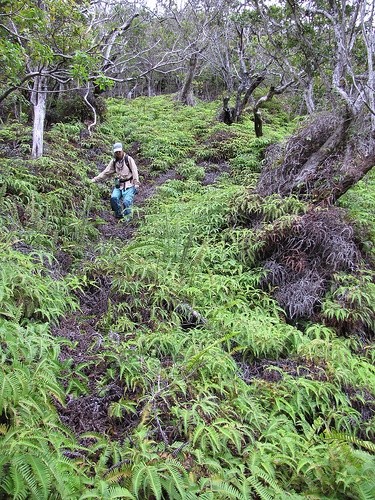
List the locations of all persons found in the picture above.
[87,143,139,220]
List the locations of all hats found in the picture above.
[113,142,123,153]
